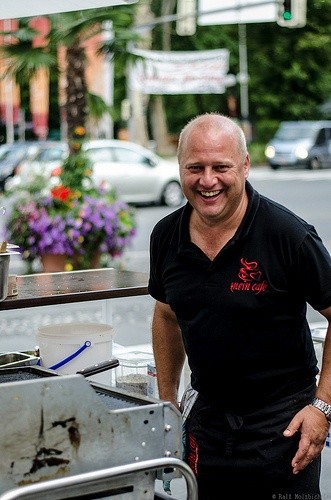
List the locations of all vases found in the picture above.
[37,249,103,272]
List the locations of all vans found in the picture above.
[264,120,331,170]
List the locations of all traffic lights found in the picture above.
[282,0,294,21]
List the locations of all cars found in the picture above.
[44,138,186,209]
[0,141,77,199]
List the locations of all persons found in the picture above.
[148,114,331,500]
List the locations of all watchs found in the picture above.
[312,397,331,423]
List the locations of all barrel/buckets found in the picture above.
[37,321,114,387]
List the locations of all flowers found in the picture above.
[5,124,139,270]
